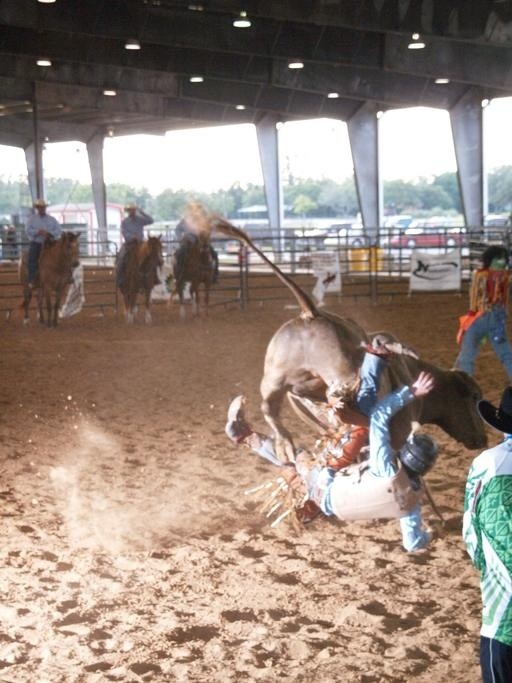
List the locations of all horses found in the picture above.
[16,230,82,328]
[113,229,165,325]
[172,229,218,313]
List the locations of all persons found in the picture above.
[117,203,153,286]
[176,202,219,285]
[453,246,512,381]
[26,199,62,289]
[462,386,512,683]
[224,333,439,552]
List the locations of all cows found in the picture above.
[180,201,490,468]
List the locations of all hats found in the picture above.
[124,205,136,210]
[483,246,510,269]
[478,387,512,432]
[34,200,49,207]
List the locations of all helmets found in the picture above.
[398,433,437,476]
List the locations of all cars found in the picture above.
[295,214,509,248]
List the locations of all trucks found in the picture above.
[45,203,124,256]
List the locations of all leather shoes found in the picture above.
[225,396,248,437]
[373,334,422,359]
